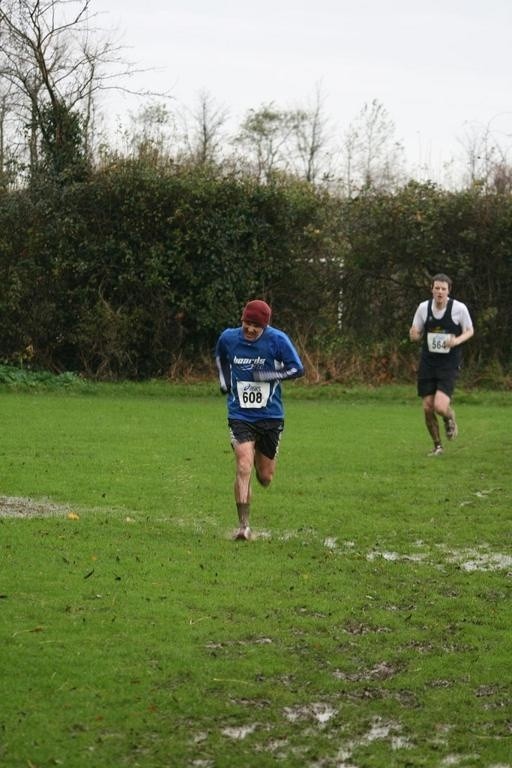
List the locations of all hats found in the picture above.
[241,299,272,329]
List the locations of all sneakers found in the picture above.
[427,445,444,456]
[234,526,252,541]
[443,416,458,441]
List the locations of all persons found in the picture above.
[215,300,305,540]
[409,273,475,455]
